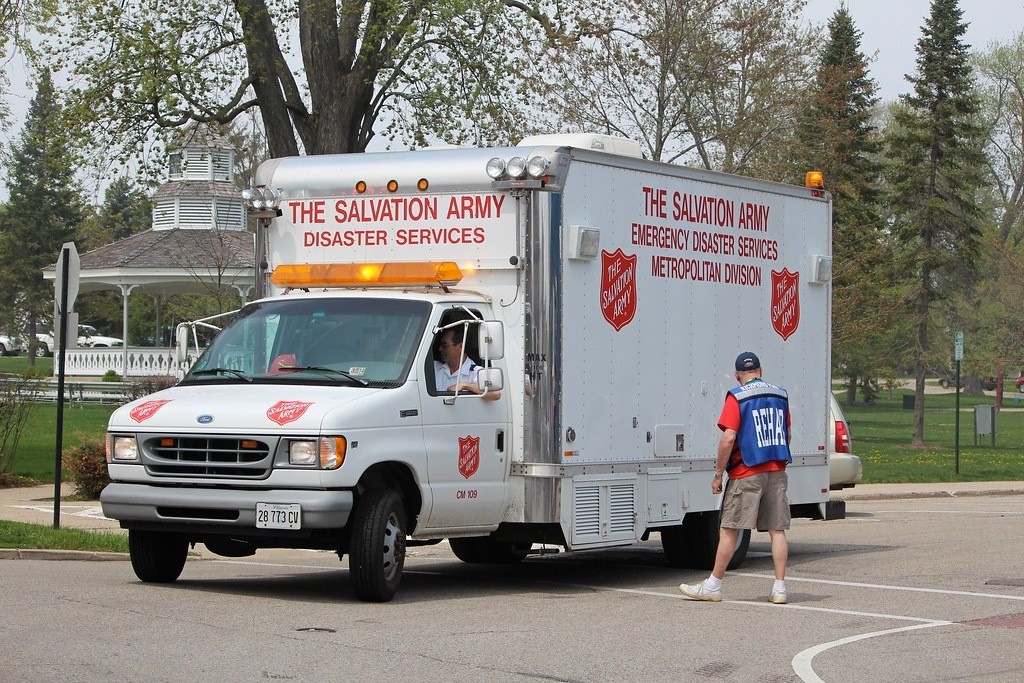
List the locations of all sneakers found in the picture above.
[768,588,787,605]
[679,579,722,602]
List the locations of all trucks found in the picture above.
[100,133,837,602]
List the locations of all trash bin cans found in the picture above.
[902,395,915,409]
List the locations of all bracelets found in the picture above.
[714,471,723,478]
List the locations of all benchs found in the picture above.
[5,377,142,404]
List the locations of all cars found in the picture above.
[828,389,863,494]
[938,371,999,392]
[1015,375,1024,393]
[0,321,123,358]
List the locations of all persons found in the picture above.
[678,351,793,604]
[432,325,501,401]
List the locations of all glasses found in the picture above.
[440,343,456,349]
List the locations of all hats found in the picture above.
[735,351,760,371]
